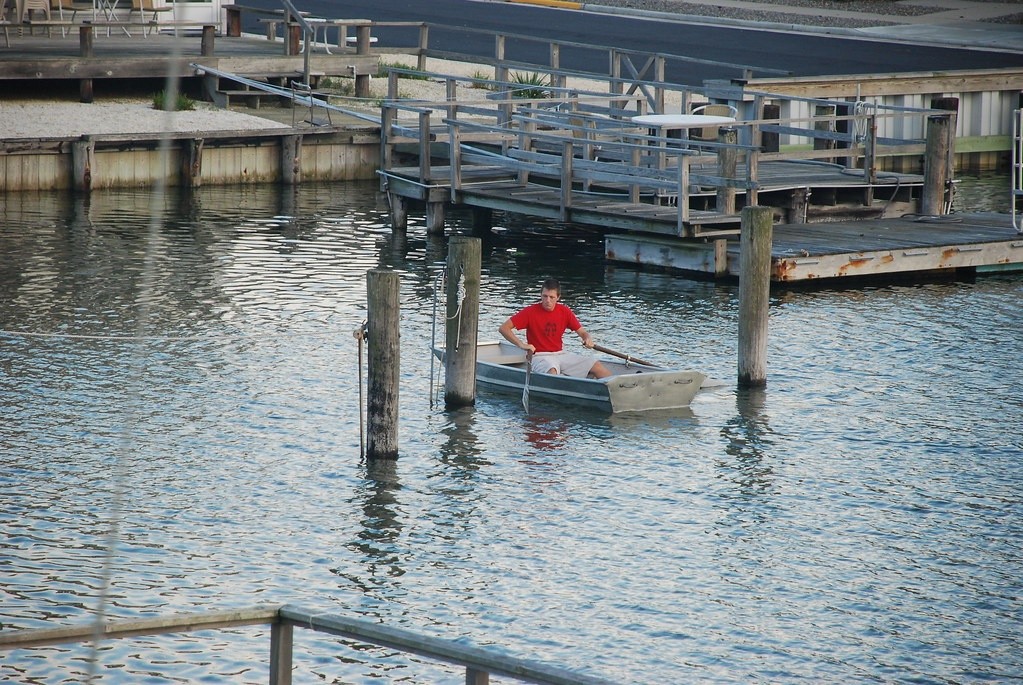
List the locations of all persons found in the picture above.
[498,278,613,380]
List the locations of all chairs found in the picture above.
[686,103,738,170]
[122,0,179,40]
[43,0,95,37]
[289,79,334,127]
[0,0,52,48]
[567,109,620,151]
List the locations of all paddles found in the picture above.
[520,349,534,415]
[581,340,661,368]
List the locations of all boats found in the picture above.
[428,339,708,415]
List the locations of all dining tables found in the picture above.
[91,0,132,39]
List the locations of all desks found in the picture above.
[300,17,334,55]
[633,113,737,187]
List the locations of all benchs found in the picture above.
[478,355,526,365]
